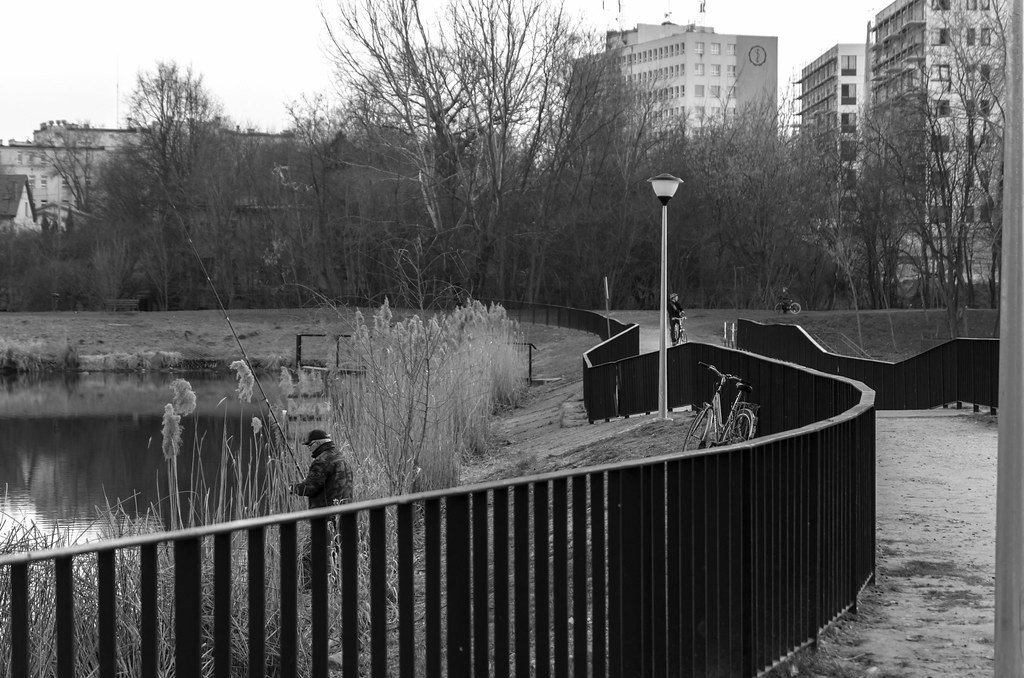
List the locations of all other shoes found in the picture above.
[672,341,676,346]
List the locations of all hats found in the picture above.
[670,293,678,299]
[301,429,330,444]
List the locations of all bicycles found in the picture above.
[774,300,802,314]
[670,316,688,345]
[683,362,757,452]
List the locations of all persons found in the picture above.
[668,293,685,346]
[781,287,791,314]
[288,428,353,590]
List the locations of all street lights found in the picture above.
[39,161,61,262]
[645,171,684,418]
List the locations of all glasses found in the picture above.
[307,441,316,448]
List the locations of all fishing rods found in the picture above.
[153,168,305,484]
